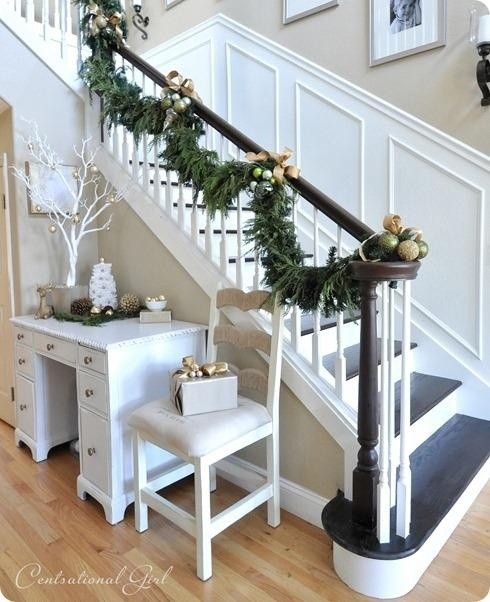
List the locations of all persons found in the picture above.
[390,0,422,33]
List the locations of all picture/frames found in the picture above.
[163,0,183,10]
[282,0,447,68]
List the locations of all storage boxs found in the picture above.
[165,368,239,419]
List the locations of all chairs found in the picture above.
[128,282,287,583]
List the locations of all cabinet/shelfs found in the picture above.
[7,315,208,526]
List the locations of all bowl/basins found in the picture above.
[146,300,168,312]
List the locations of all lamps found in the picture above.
[130,0,150,39]
[469,8,490,106]
